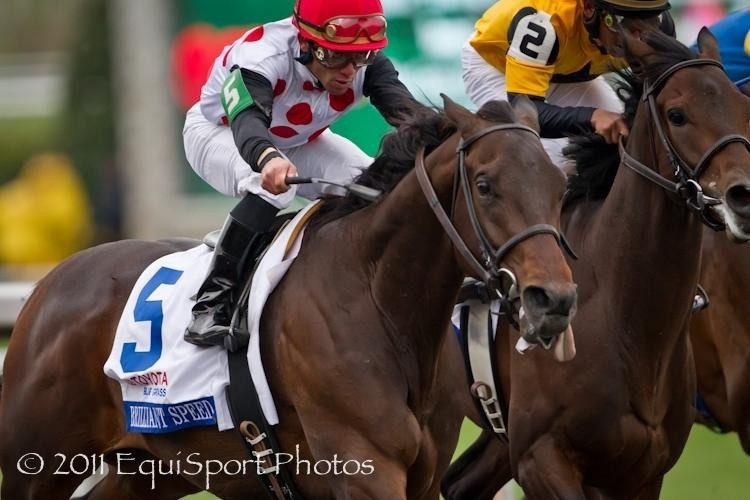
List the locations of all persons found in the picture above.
[457,0,674,184]
[180,0,440,347]
[687,10,749,98]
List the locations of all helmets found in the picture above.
[292,0,388,51]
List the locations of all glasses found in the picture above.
[324,14,387,44]
[310,41,379,68]
[604,13,663,32]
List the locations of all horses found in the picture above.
[0,89,584,500]
[430,24,750,500]
[688,220,750,457]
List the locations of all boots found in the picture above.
[184,214,266,346]
